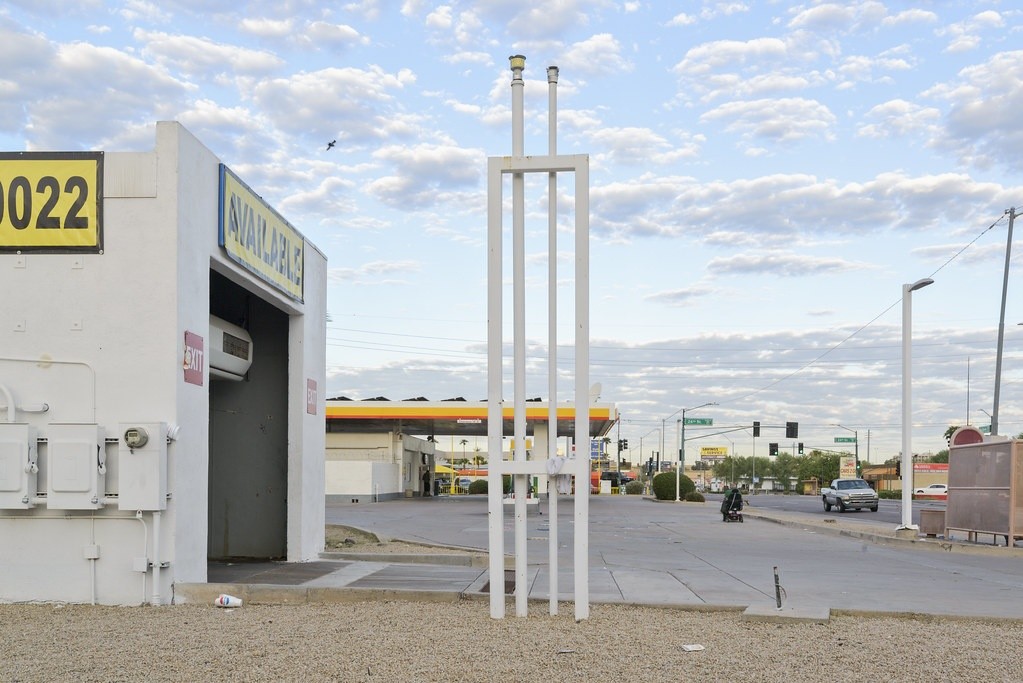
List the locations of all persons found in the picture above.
[422,470,431,497]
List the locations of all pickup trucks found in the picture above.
[821,478,879,513]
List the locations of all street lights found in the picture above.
[837,424,859,478]
[735,423,754,495]
[895,277,935,539]
[681,402,719,474]
[977,408,993,425]
[640,428,661,465]
[674,419,682,502]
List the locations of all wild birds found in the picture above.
[326,140,336,151]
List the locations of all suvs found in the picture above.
[600,471,636,487]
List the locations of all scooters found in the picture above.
[723,497,743,522]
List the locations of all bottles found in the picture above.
[220,594,243,607]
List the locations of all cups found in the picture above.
[214,596,233,607]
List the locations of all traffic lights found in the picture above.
[798,443,804,454]
[618,439,623,451]
[623,439,628,450]
[769,443,778,456]
[622,458,626,465]
[856,461,860,471]
[895,460,900,476]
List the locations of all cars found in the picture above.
[455,477,471,487]
[912,484,948,494]
